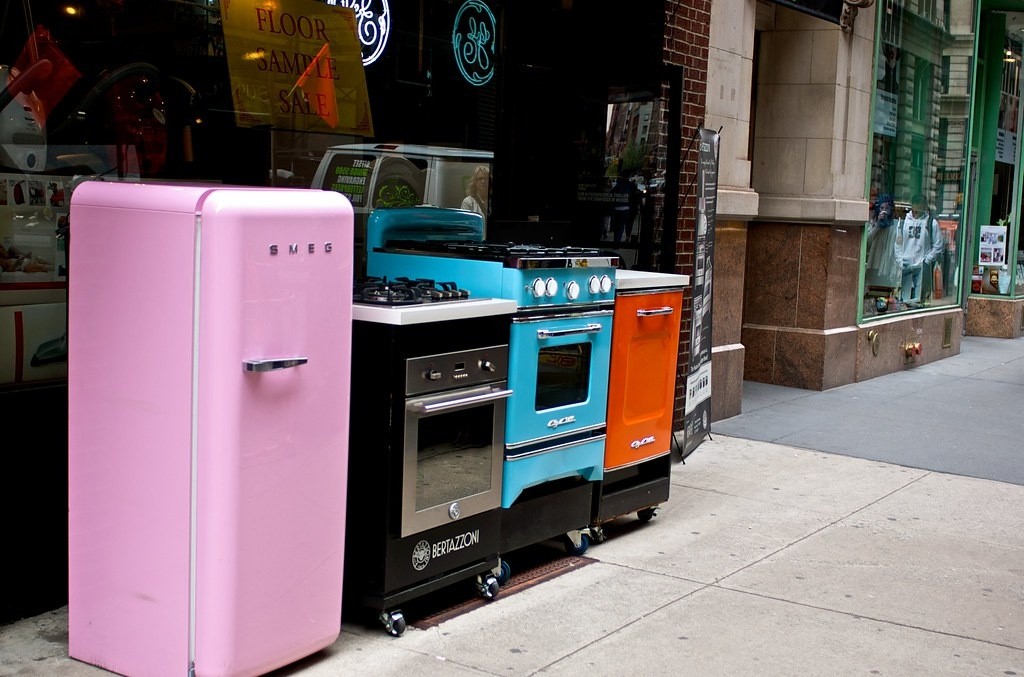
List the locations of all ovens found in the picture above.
[502,308,613,584]
[354,317,514,636]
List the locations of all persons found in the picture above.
[895,194,943,310]
[0,245,54,271]
[954,222,961,267]
[863,196,903,314]
[50,183,64,206]
[34,188,45,203]
[461,166,489,239]
[940,243,950,298]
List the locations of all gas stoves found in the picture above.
[373,238,620,270]
[353,275,470,308]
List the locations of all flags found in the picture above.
[298,43,339,128]
